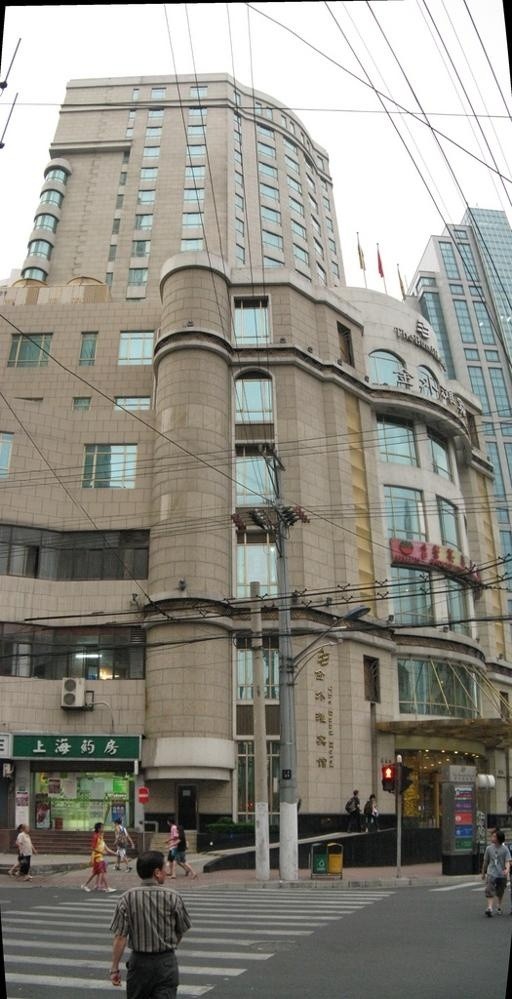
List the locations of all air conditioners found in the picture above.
[61,676,87,708]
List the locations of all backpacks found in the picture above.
[345,798,358,813]
[364,801,374,816]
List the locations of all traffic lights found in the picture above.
[380,763,395,793]
[401,765,414,793]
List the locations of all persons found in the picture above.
[107,849,192,999]
[80,822,120,894]
[163,817,191,876]
[481,830,512,917]
[112,819,135,872]
[166,825,197,880]
[345,790,362,832]
[363,794,380,833]
[7,823,38,882]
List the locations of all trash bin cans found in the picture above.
[309,842,344,879]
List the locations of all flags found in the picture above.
[357,242,366,270]
[397,268,406,300]
[377,245,384,278]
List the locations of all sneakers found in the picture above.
[484,907,502,918]
[116,865,133,873]
[82,881,116,894]
[8,870,33,881]
[166,870,198,880]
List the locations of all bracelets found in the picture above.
[108,969,120,974]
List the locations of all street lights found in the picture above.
[276,602,374,881]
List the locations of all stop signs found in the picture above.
[137,786,150,805]
